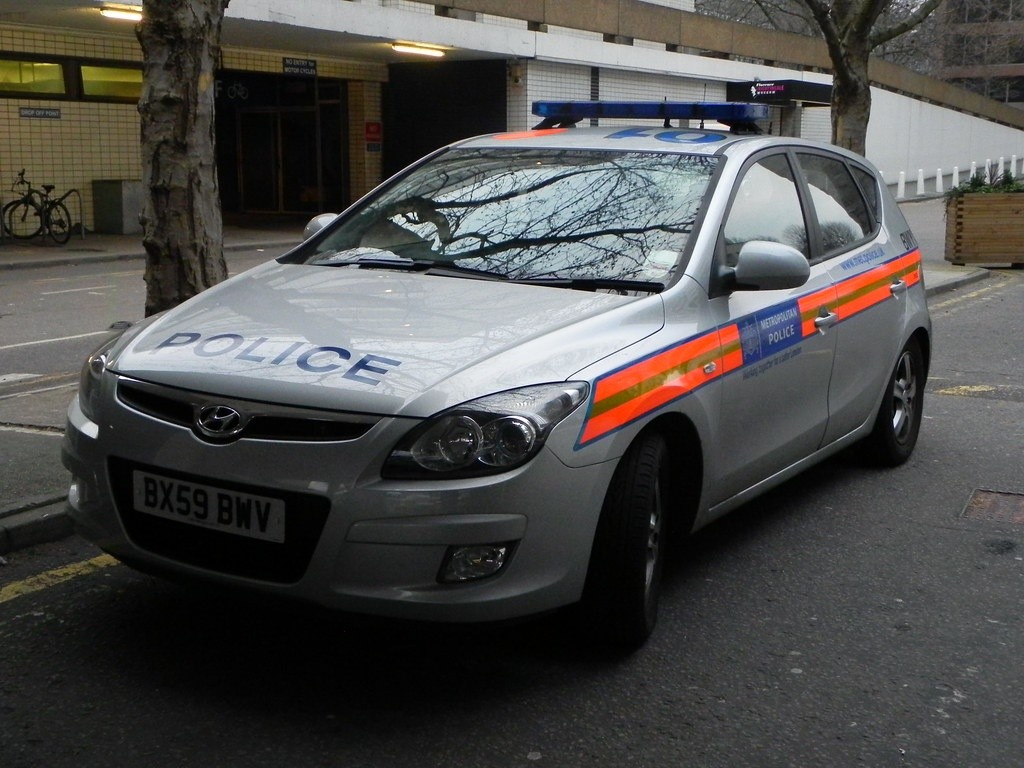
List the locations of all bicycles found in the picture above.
[0,167,72,246]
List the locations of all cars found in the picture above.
[57,98,940,651]
